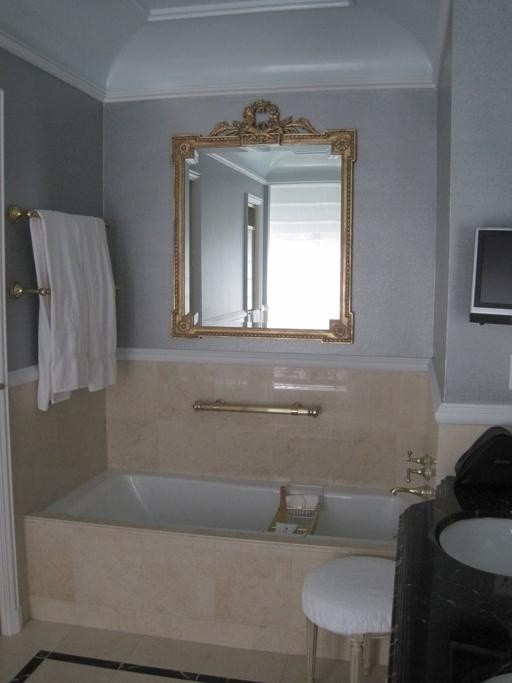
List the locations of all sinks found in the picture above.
[434,509,512,578]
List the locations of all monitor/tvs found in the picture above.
[470,227,512,324]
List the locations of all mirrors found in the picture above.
[170,98,354,343]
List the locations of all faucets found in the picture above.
[386,484,428,499]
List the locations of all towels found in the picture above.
[29,209,119,413]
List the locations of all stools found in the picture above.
[301,555,396,683]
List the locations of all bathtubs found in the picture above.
[35,466,429,545]
